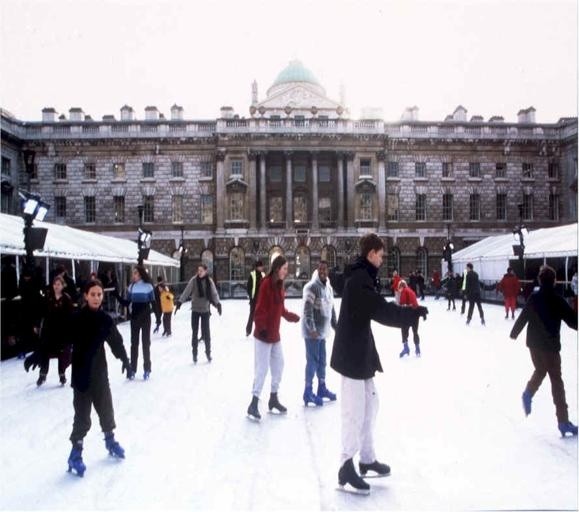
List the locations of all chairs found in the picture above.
[193,353,198,363]
[268,392,287,412]
[522,388,534,415]
[103,434,125,459]
[246,395,261,418]
[359,460,390,474]
[37,369,47,385]
[144,371,149,381]
[303,388,323,405]
[66,448,86,475]
[130,371,135,381]
[58,368,67,384]
[335,458,370,490]
[415,344,421,354]
[558,422,578,434]
[205,351,213,361]
[400,343,410,358]
[318,383,336,400]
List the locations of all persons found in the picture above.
[495,267,521,319]
[151,276,165,333]
[397,279,421,358]
[157,283,175,336]
[101,269,114,288]
[328,232,428,489]
[173,263,222,363]
[466,263,485,325]
[300,261,338,406]
[431,267,441,300]
[23,278,133,474]
[509,265,578,437]
[245,261,266,337]
[416,271,426,301]
[246,254,301,419]
[113,265,161,381]
[81,272,103,311]
[408,272,417,296]
[570,265,578,309]
[5,263,79,387]
[392,270,400,296]
[443,272,456,311]
[459,269,468,314]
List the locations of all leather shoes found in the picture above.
[153,322,160,331]
[111,289,119,297]
[174,302,181,315]
[24,351,38,372]
[217,304,221,315]
[122,359,130,379]
[414,305,428,324]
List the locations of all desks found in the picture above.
[136,204,152,266]
[513,222,528,279]
[178,225,188,283]
[21,148,51,266]
[443,243,455,276]
